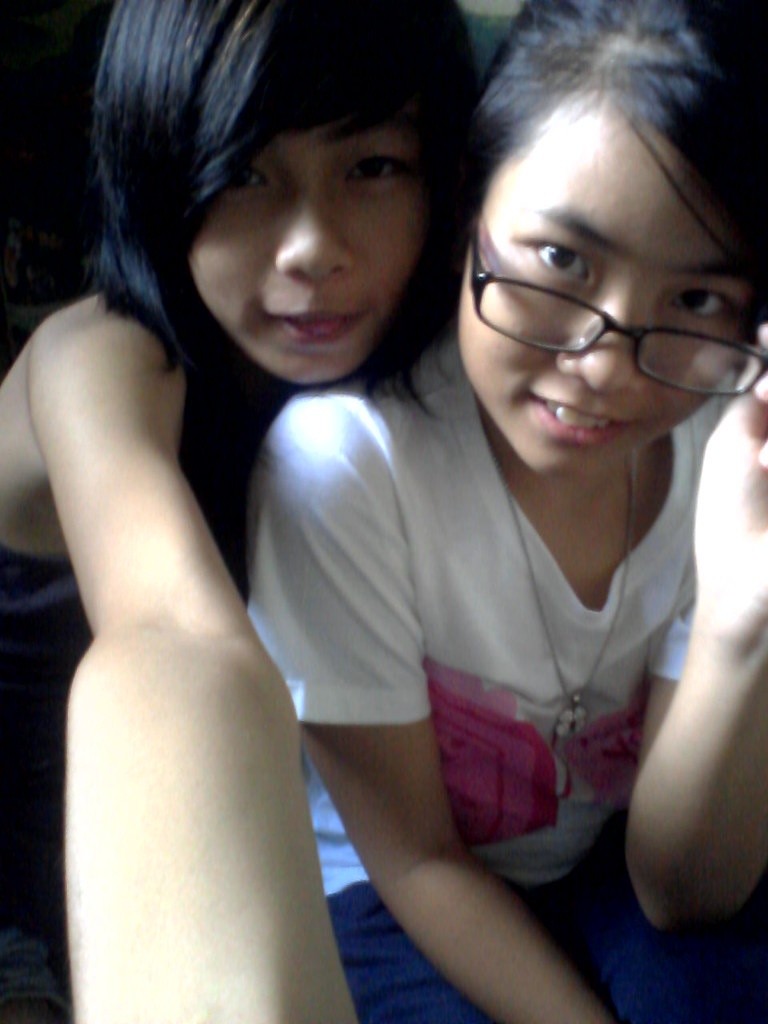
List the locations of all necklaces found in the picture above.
[491,451,637,741]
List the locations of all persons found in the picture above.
[0,0,768,1024]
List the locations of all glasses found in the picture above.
[466,198,768,396]
